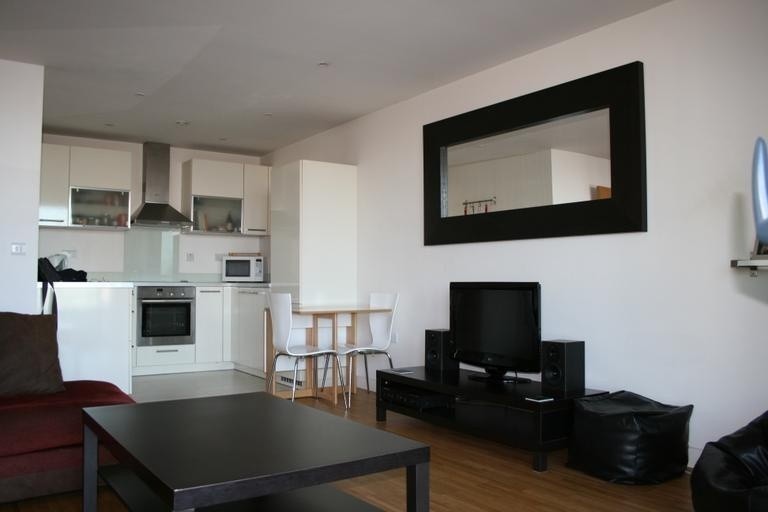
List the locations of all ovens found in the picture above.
[134,285,196,346]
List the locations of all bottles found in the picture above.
[102,212,111,225]
[226,213,234,232]
[199,212,208,231]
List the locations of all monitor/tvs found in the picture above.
[450,282,541,383]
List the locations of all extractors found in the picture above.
[131,141,195,231]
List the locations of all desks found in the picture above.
[287,303,391,404]
[79,388,432,512]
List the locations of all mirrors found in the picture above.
[423,60,650,244]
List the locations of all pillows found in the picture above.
[1,312,63,399]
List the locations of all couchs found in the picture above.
[0,378,148,503]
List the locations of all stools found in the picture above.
[571,387,694,485]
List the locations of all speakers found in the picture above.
[425,328,459,375]
[541,339,585,400]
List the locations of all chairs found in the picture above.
[267,290,350,410]
[335,287,403,416]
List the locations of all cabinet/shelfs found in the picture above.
[232,288,267,372]
[38,140,132,230]
[182,158,268,236]
[195,286,231,367]
[266,160,360,306]
[38,281,132,399]
[135,346,195,368]
[373,361,611,478]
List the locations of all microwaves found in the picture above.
[223,256,263,282]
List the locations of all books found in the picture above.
[524,394,555,403]
